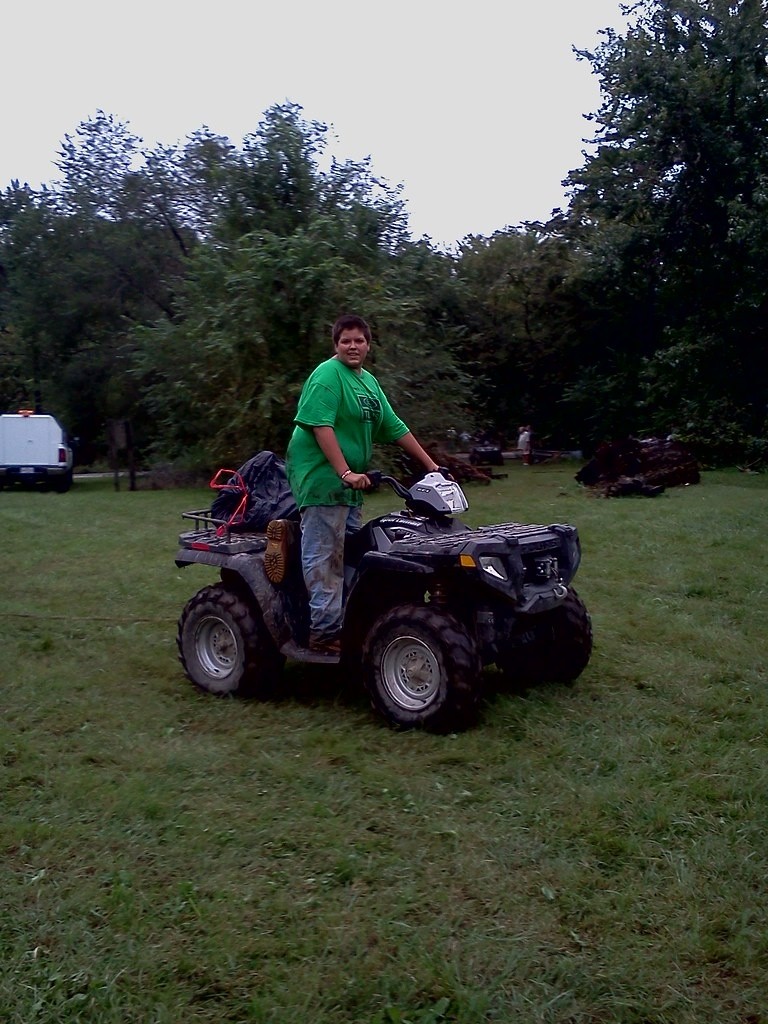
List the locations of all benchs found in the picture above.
[177,507,269,555]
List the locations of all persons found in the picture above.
[517,426,530,465]
[286,316,454,654]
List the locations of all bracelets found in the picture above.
[340,470,351,479]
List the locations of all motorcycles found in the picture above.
[175,450,591,732]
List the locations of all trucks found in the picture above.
[0,410,75,496]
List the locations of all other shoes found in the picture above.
[309,632,342,657]
[265,519,288,583]
